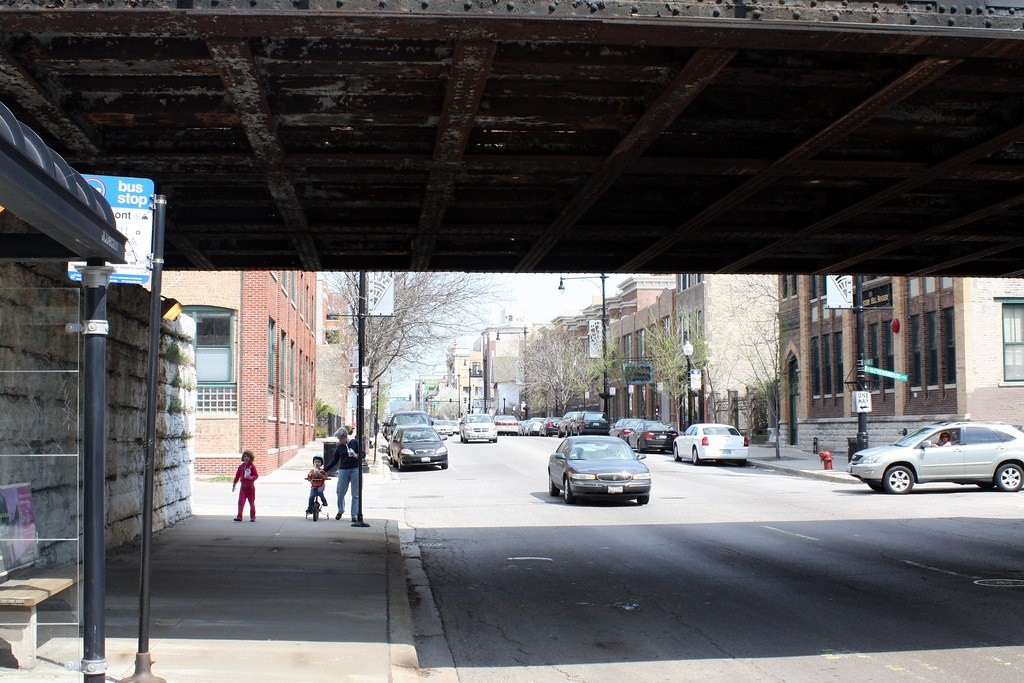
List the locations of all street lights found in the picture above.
[443,374,461,417]
[496,327,529,420]
[682,340,697,425]
[558,273,610,424]
[451,367,472,415]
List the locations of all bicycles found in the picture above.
[305,476,332,522]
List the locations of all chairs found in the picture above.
[574,447,586,457]
[951,431,960,445]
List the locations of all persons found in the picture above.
[324,427,366,522]
[232,450,258,522]
[305,456,327,514]
[931,432,951,447]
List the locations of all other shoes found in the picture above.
[352,517,357,521]
[251,518,255,522]
[306,508,312,514]
[234,518,242,521]
[323,499,328,506]
[336,512,342,520]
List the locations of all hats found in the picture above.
[313,456,324,466]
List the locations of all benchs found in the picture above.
[0,565,84,669]
[584,452,604,458]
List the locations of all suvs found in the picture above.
[383,410,436,441]
[557,411,580,437]
[571,411,611,435]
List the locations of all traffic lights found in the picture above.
[409,395,413,402]
[466,404,470,414]
[450,399,452,403]
[463,397,467,403]
[426,398,429,403]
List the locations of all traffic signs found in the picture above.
[854,390,873,413]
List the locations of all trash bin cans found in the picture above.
[323,442,339,476]
[848,437,859,462]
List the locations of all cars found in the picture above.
[673,424,750,466]
[388,425,450,468]
[539,416,563,438]
[626,419,681,453]
[547,435,653,504]
[519,416,547,436]
[846,422,1024,495]
[450,421,460,434]
[495,415,520,435]
[459,413,499,444]
[433,419,453,436]
[608,417,647,443]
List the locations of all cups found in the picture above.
[245,469,251,478]
[314,469,321,477]
[348,449,354,457]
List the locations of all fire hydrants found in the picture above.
[819,451,833,470]
